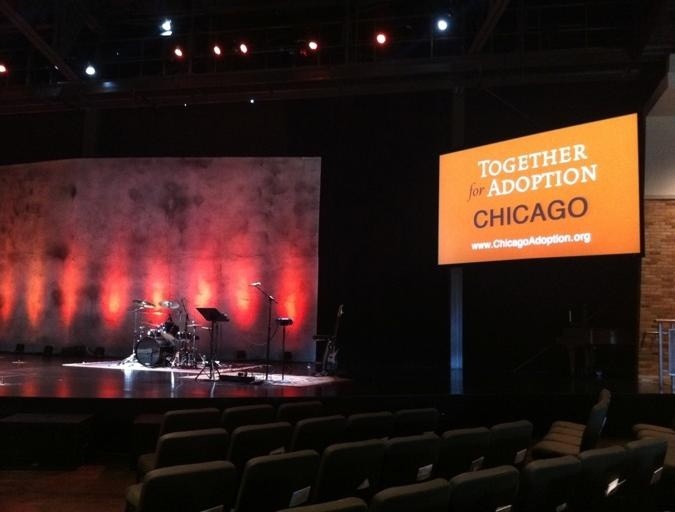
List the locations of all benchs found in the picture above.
[119,399,674,512]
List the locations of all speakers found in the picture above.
[62,345,88,358]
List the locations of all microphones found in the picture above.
[249,282,262,287]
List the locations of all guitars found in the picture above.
[322,304,347,376]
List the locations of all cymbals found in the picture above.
[187,324,200,327]
[202,327,211,330]
[159,300,180,309]
[132,299,155,308]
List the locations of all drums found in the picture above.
[176,347,198,369]
[164,322,179,337]
[135,335,177,368]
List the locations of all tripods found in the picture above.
[117,313,141,365]
[194,322,221,382]
